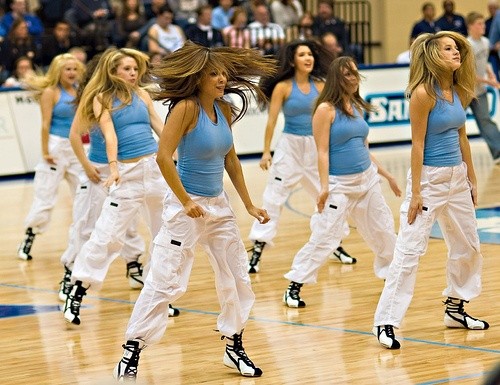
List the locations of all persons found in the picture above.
[283,57,402,307]
[249,38,356,272]
[482,0,500,83]
[411,0,468,43]
[0,0,352,87]
[63,48,180,326]
[372,32,489,349]
[60,57,146,300]
[466,11,500,163]
[112,42,270,385]
[19,53,90,260]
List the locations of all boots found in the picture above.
[115,338,148,383]
[18,227,36,259]
[329,247,357,264]
[59,266,72,302]
[62,281,90,325]
[441,297,489,329]
[372,325,401,350]
[216,329,262,377]
[282,281,305,308]
[126,256,145,288]
[168,304,179,317]
[247,240,265,273]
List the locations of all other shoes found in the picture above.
[493,155,500,164]
